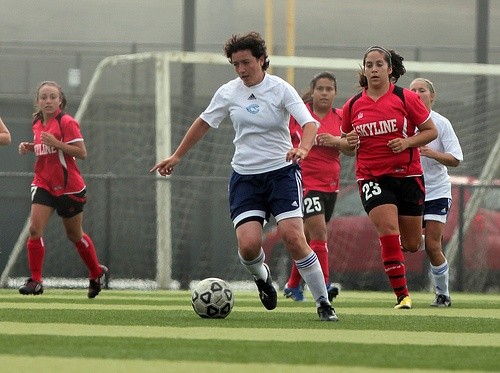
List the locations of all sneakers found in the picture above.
[252,264,278,312]
[87,265,109,299]
[430,293,451,308]
[316,300,340,321]
[394,296,414,310]
[327,282,341,300]
[17,277,45,296]
[283,282,304,301]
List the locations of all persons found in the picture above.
[0,117,11,145]
[150,31,338,320]
[282,72,345,304]
[18,81,109,298]
[400,76,463,308]
[339,45,438,309]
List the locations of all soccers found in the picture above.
[191,278,234,320]
